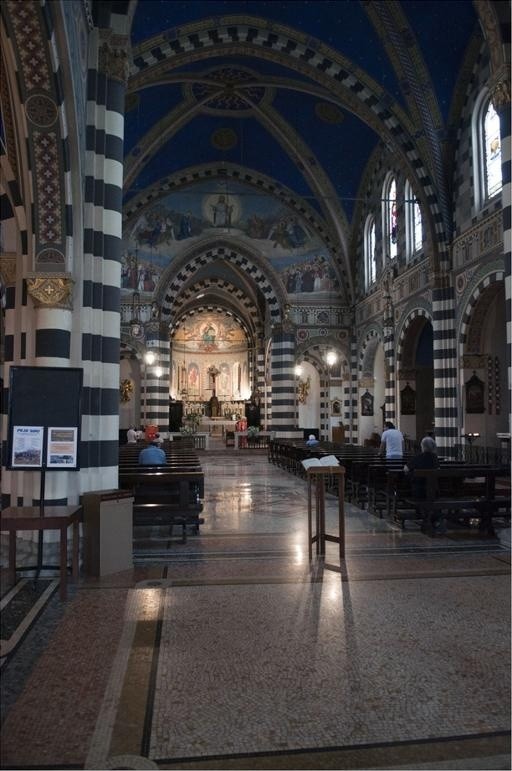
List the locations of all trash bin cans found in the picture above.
[81,488,135,577]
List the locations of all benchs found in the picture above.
[268,438,512,538]
[119,441,205,543]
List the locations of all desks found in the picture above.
[173,435,206,449]
[239,435,270,450]
[0,506,83,600]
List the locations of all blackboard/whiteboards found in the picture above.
[6,365,84,471]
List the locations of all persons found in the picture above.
[377,421,405,458]
[138,442,167,465]
[306,434,319,447]
[121,194,338,296]
[126,424,146,444]
[404,437,440,471]
[152,433,164,444]
[238,416,248,448]
